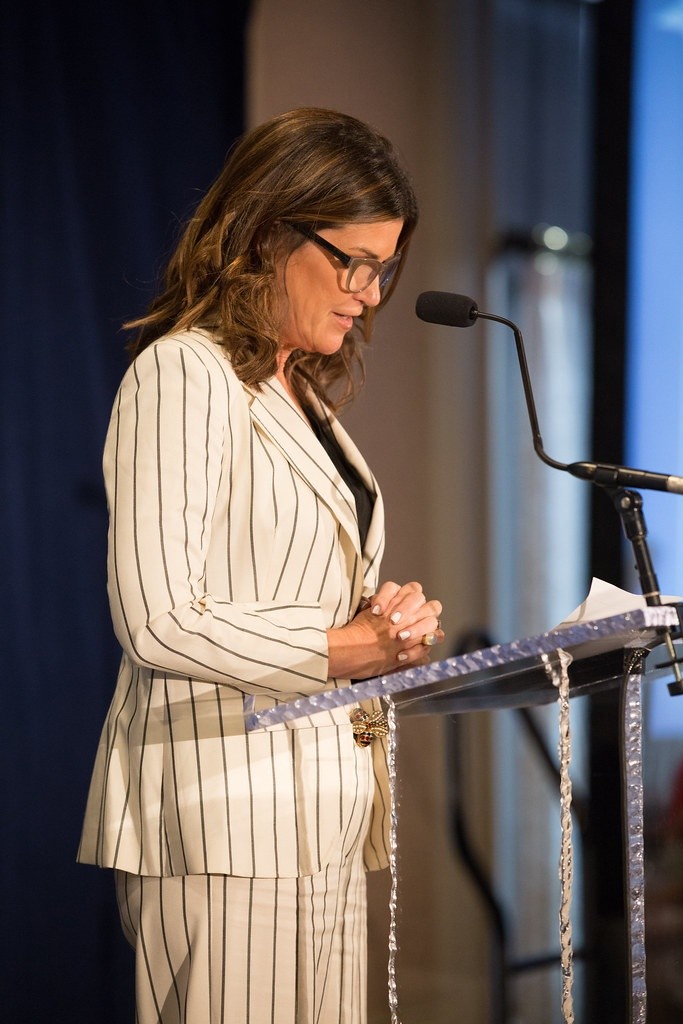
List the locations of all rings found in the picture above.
[422,633,437,646]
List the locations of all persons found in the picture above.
[74,104,445,1024]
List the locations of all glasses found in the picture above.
[282,220,404,294]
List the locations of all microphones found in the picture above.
[414,290,682,494]
